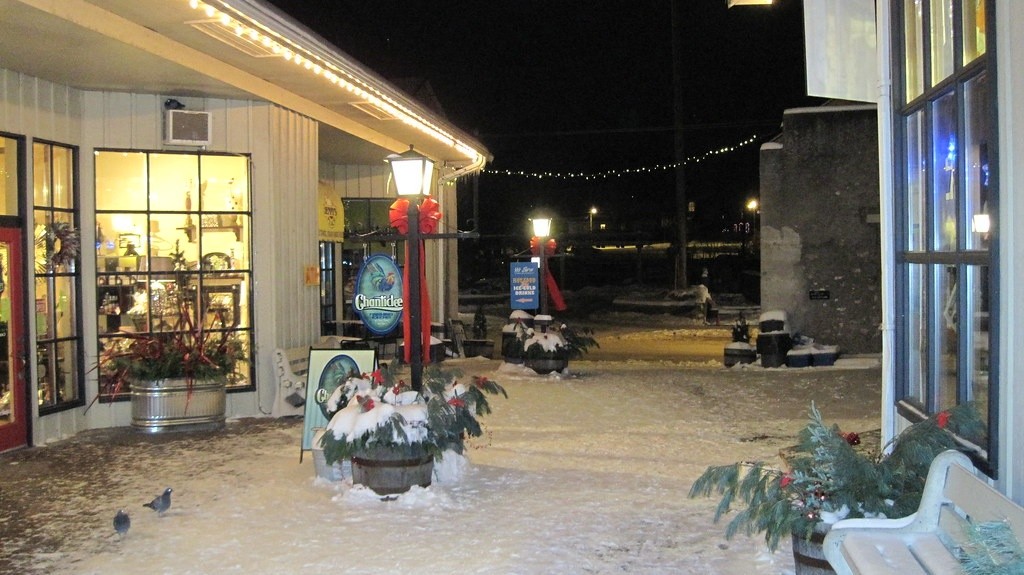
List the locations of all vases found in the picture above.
[442,431,463,456]
[312,447,351,480]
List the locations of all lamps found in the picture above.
[137,255,178,332]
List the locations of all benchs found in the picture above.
[272,337,341,421]
[821,449,1024,575]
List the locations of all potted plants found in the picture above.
[687,397,987,575]
[83,284,261,433]
[462,305,601,375]
[314,355,509,503]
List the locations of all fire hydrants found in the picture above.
[472,306,488,340]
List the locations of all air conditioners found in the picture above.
[163,109,212,146]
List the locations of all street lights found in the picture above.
[749,201,756,242]
[589,208,598,234]
